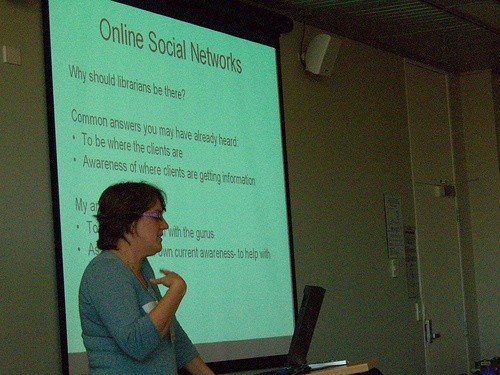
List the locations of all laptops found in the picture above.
[253,285,327,375]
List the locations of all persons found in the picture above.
[78,181,215,375]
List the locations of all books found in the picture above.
[307,358,376,375]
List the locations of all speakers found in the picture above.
[304,33,344,77]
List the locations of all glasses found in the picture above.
[141,211,165,222]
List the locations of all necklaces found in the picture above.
[119,250,159,305]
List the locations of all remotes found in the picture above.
[308,359,350,370]
